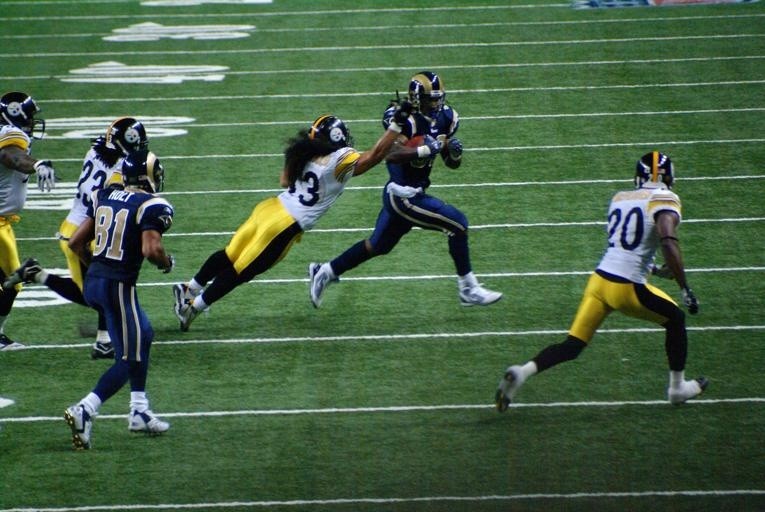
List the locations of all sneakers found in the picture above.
[91,341,125,360]
[458,284,505,307]
[127,409,171,433]
[667,376,709,406]
[2,258,43,289]
[494,363,528,414]
[307,260,331,309]
[0,333,26,351]
[172,283,200,334]
[64,404,93,454]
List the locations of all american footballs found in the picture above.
[406,135,435,148]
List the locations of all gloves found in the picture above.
[651,263,679,281]
[423,134,442,157]
[33,160,62,193]
[392,101,414,129]
[447,138,463,158]
[681,288,700,315]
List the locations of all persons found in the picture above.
[0,91,63,349]
[308,72,503,308]
[494,151,710,412]
[0,118,150,359]
[63,149,174,450]
[173,101,412,332]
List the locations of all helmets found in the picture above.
[408,71,448,120]
[307,114,348,149]
[121,150,164,193]
[634,151,676,186]
[1,92,46,140]
[105,117,147,152]
